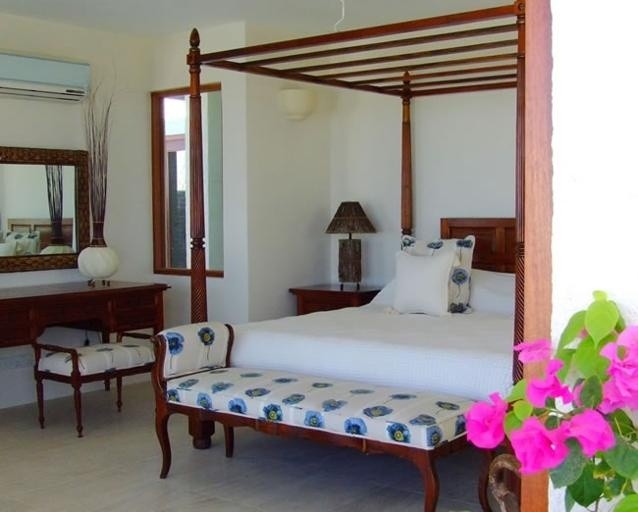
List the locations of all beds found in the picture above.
[1,217,75,260]
[183,0,531,453]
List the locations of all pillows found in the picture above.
[7,230,43,254]
[390,250,457,317]
[396,234,478,314]
[2,240,20,255]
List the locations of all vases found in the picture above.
[39,231,74,255]
[77,220,121,281]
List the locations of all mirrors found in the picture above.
[0,143,92,275]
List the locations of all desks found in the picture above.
[1,282,170,385]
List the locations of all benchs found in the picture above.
[151,319,484,512]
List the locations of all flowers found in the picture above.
[461,288,637,512]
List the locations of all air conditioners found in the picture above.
[0,51,96,104]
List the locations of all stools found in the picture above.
[27,324,155,439]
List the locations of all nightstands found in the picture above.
[291,280,380,315]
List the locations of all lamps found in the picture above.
[324,199,377,290]
